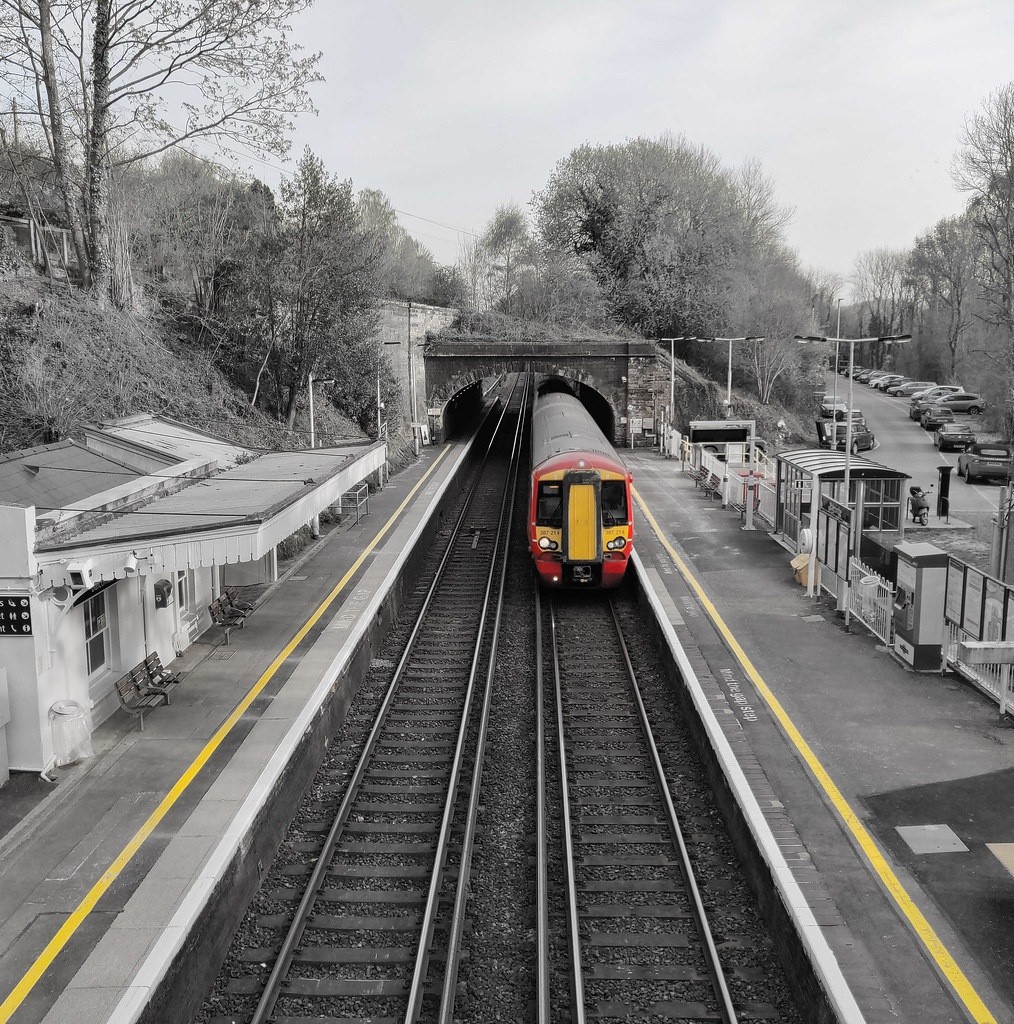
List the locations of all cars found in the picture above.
[910,399,941,421]
[934,423,977,452]
[813,384,874,455]
[828,353,912,392]
[921,407,956,430]
[957,444,1014,484]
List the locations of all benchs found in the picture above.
[113,651,190,731]
[208,585,259,646]
[698,473,721,503]
[734,495,761,526]
[687,465,710,492]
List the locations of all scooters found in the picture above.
[910,483,934,526]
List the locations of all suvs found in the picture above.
[887,382,937,397]
[911,386,986,416]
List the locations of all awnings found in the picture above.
[33,438,390,585]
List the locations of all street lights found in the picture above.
[650,335,697,459]
[414,343,431,457]
[309,375,335,538]
[697,336,766,509]
[377,342,401,489]
[830,297,845,450]
[793,335,913,509]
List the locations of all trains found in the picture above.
[527,372,633,591]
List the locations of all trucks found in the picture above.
[690,427,768,462]
